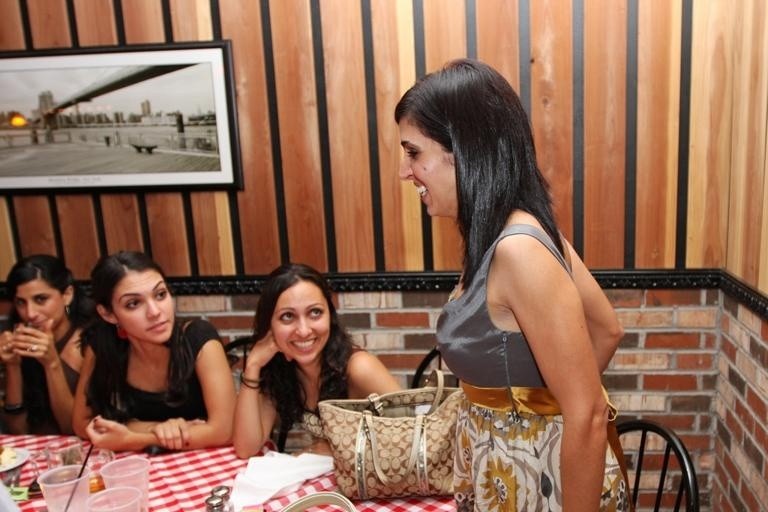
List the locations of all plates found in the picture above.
[0,445,29,473]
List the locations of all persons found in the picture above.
[0,253,94,435]
[70,249,239,452]
[231,260,405,459]
[394,58,642,511]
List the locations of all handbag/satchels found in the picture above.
[317,369,466,500]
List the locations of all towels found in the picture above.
[221,448,335,512]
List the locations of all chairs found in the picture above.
[223,336,259,395]
[616,419,699,511]
[411,345,458,405]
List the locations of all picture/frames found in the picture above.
[0,39,244,196]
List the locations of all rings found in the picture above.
[308,447,313,452]
[29,342,38,352]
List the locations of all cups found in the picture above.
[36,436,152,512]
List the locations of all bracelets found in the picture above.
[4,403,26,415]
[240,377,262,390]
[240,371,262,384]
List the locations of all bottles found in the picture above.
[204,485,232,511]
